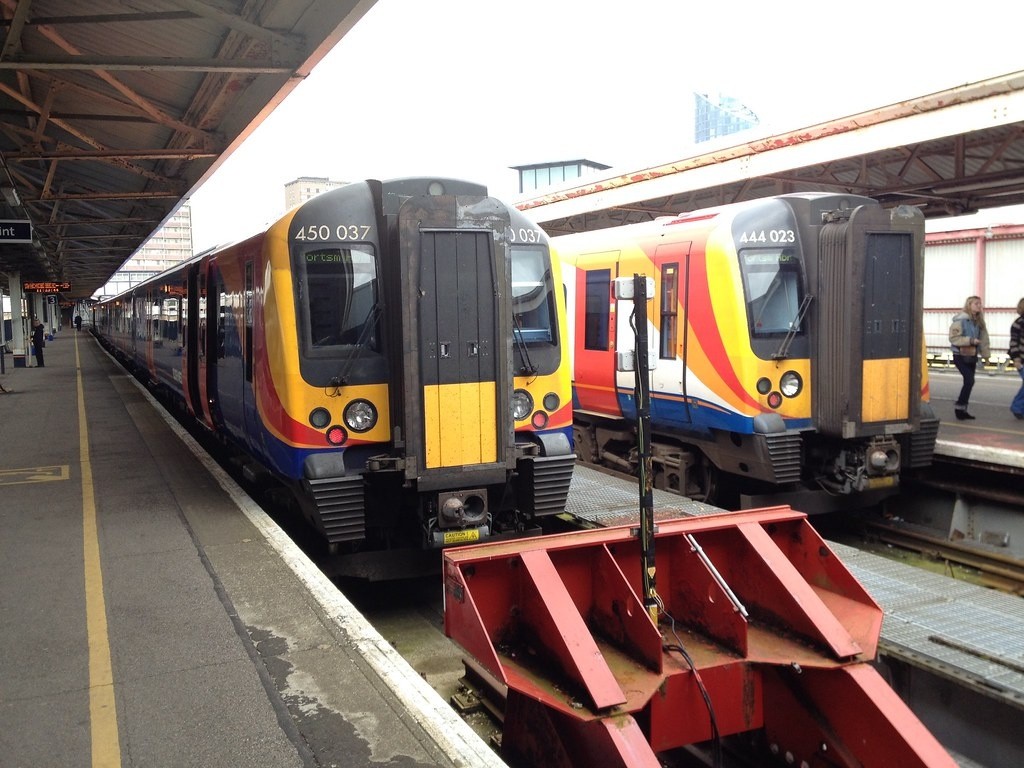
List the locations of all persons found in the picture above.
[1007,298,1024,420]
[74,313,82,331]
[948,296,991,422]
[33,319,44,368]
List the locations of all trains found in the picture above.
[89,177,578,579]
[549,191,940,516]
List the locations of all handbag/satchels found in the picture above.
[959,345,977,357]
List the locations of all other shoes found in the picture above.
[1009,405,1024,421]
[35,365,45,368]
[955,408,977,421]
